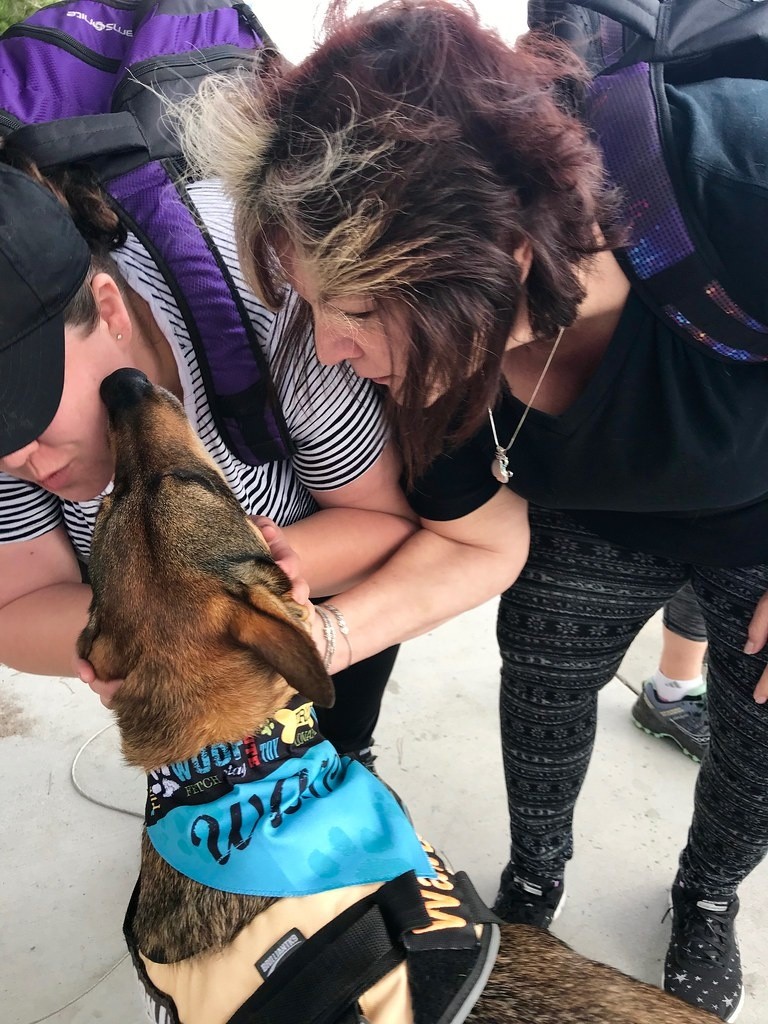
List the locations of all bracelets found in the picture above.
[314,604,335,673]
[320,605,352,668]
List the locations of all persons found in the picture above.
[0,161,416,764]
[218,0,768,1022]
[631,582,713,763]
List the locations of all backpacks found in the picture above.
[528,0,768,361]
[0,0,295,464]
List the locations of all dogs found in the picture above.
[73,366,732,1023]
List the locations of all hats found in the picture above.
[0,161,91,458]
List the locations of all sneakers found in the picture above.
[491,862,564,932]
[661,866,744,1022]
[631,678,711,762]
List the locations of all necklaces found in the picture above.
[488,325,565,484]
